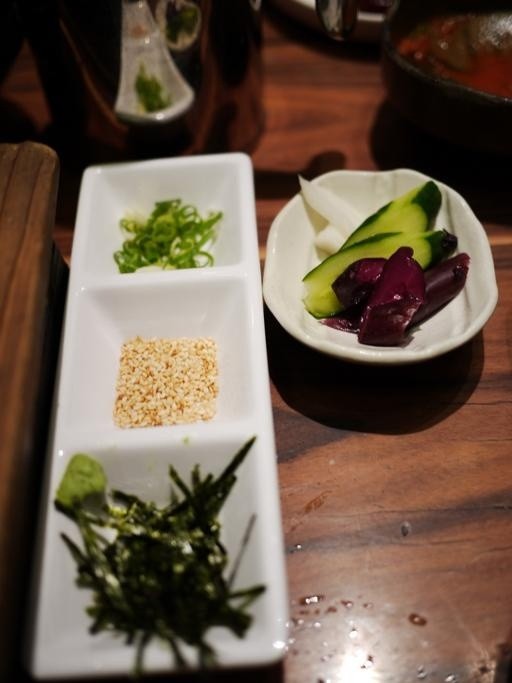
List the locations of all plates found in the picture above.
[262,169,498,365]
[29,149,291,683]
[155,0,203,52]
[113,0,196,125]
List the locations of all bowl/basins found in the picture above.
[377,0,511,152]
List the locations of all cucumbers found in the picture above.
[339,180,443,250]
[303,228,459,320]
